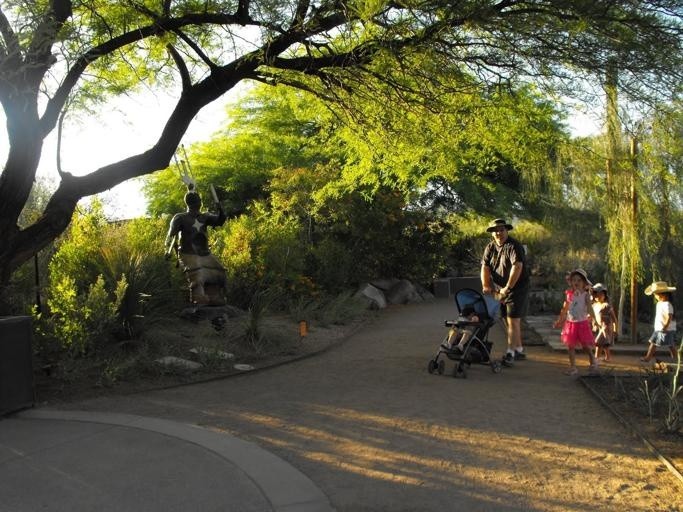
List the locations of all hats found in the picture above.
[565,268,592,290]
[644,282,676,296]
[589,283,607,301]
[486,220,512,232]
[461,307,475,316]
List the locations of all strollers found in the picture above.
[427,288,511,377]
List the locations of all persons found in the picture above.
[587,282,618,363]
[163,189,228,306]
[639,280,679,363]
[480,218,531,366]
[439,308,480,353]
[553,267,602,376]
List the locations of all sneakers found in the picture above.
[514,348,526,361]
[440,342,451,350]
[589,361,596,370]
[501,353,514,367]
[565,368,577,376]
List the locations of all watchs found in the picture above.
[505,285,512,290]
